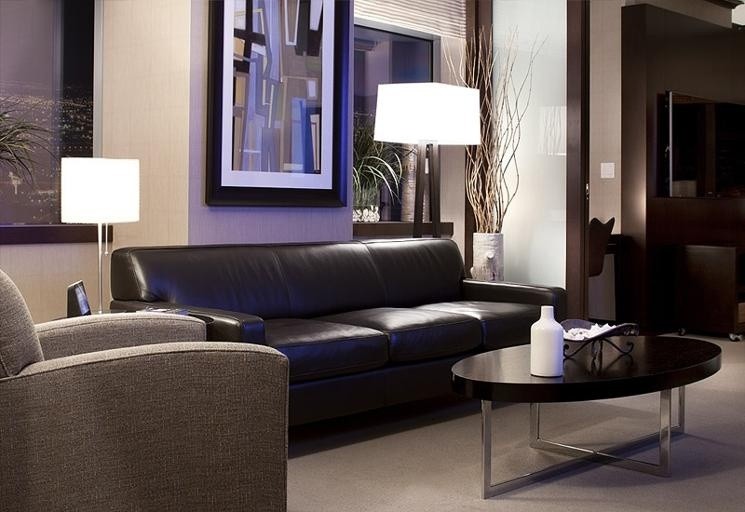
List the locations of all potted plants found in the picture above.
[353,111,417,223]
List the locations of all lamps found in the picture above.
[373,82,483,239]
[59,156,141,313]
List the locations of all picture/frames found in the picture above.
[206,0,352,209]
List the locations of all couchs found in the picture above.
[108,237,569,428]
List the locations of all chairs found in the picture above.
[0,264,290,512]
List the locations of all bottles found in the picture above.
[530,305,565,378]
